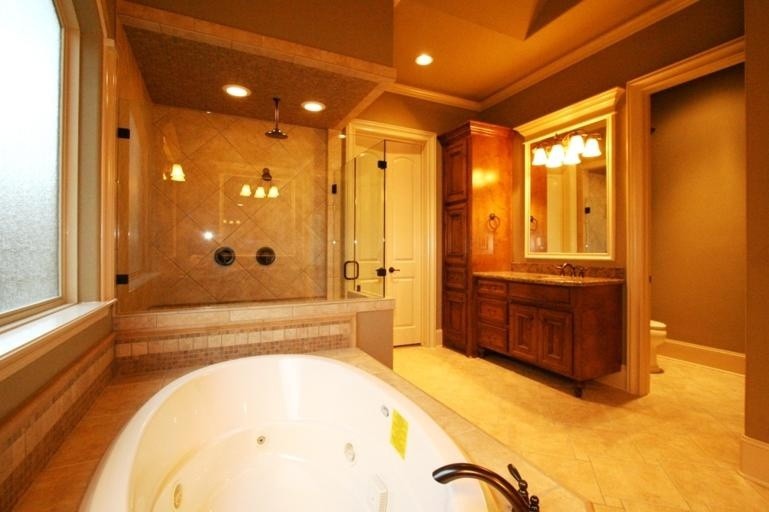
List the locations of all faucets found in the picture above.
[432,463,540,512]
[559,262,576,277]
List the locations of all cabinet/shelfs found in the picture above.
[440,122,512,356]
[474,276,507,353]
[507,280,624,398]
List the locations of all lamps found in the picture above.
[265,96,288,139]
[170,162,186,182]
[532,128,603,169]
[239,169,279,200]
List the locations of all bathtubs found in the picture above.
[77,352,488,512]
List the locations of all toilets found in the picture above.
[649,319,668,373]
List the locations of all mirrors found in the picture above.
[521,115,619,261]
